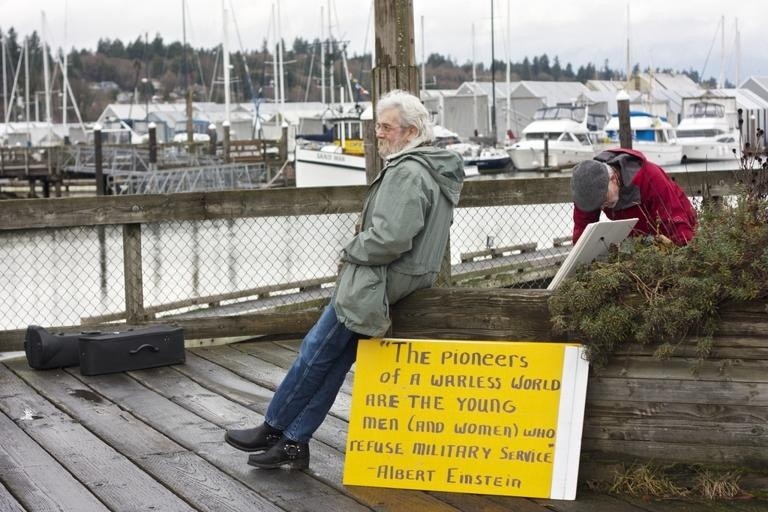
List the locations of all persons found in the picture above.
[570,148,699,247]
[224,90,465,470]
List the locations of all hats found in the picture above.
[570,161,609,213]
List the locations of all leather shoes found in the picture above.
[224,423,310,469]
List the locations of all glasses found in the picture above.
[374,124,401,130]
